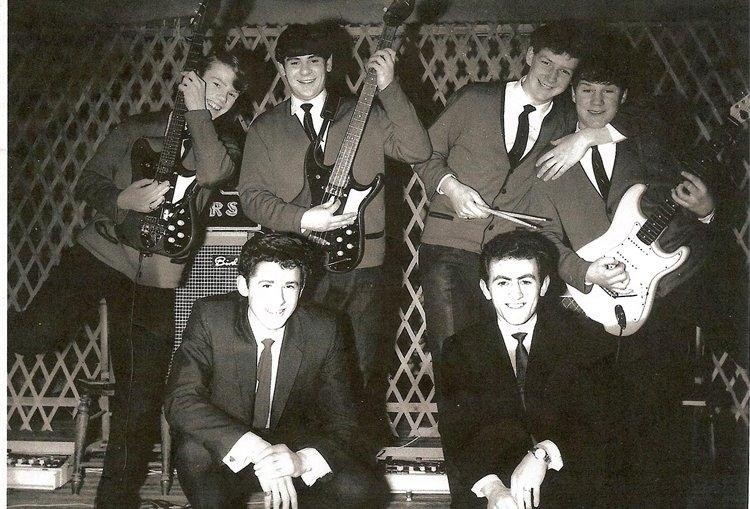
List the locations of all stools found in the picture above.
[70,375,175,496]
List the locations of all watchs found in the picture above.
[525,445,555,466]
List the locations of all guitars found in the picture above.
[113,1,220,259]
[287,0,415,273]
[566,91,750,336]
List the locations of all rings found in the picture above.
[149,204,155,212]
[263,489,273,497]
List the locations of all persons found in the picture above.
[159,234,393,509]
[525,54,724,508]
[409,22,668,356]
[8,47,256,509]
[236,19,436,468]
[432,229,630,508]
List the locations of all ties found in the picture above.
[508,104,536,169]
[251,338,275,430]
[590,145,610,199]
[300,103,316,143]
[512,332,530,410]
